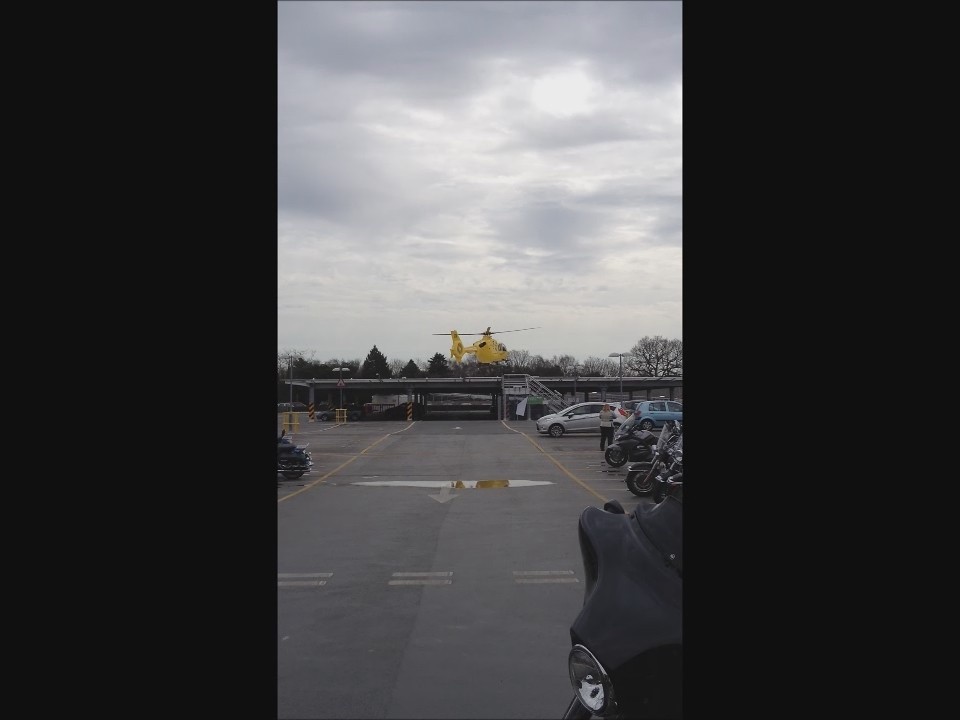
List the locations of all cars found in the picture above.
[318,399,334,411]
[613,399,660,416]
[316,406,361,421]
[277,402,307,411]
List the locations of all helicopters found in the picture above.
[432,327,542,364]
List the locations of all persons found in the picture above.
[599,403,617,450]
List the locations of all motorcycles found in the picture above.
[277,429,314,479]
[627,420,683,504]
[559,495,686,720]
[605,413,659,468]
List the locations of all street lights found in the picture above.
[278,354,304,432]
[608,352,633,408]
[332,368,350,423]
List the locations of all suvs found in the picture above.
[536,402,629,438]
[385,402,425,419]
[635,400,683,431]
